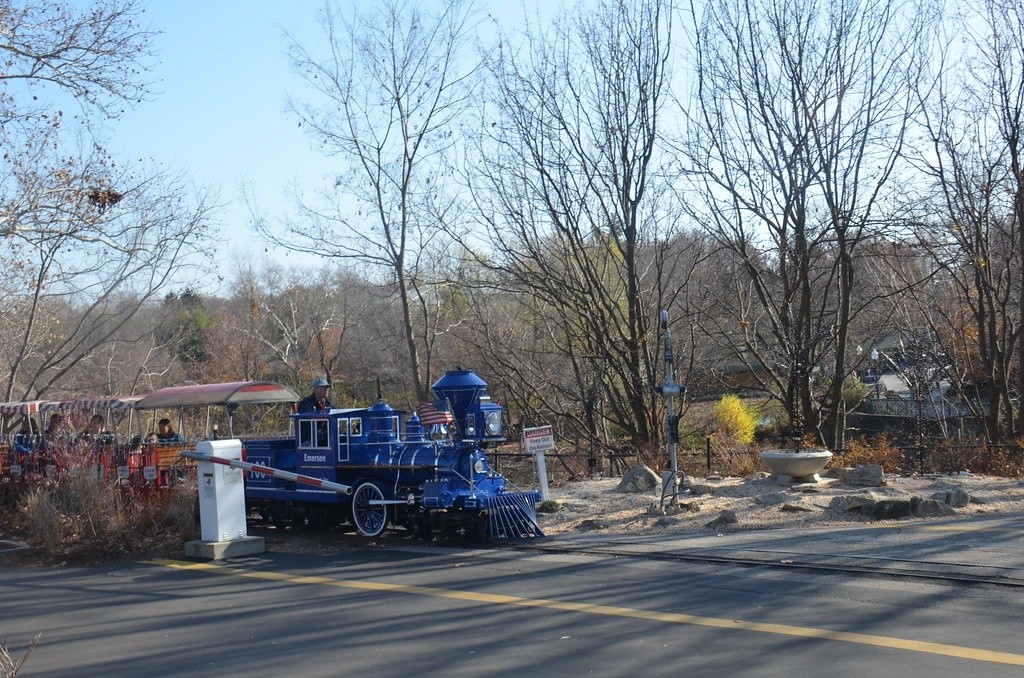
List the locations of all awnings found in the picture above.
[39,395,143,440]
[0,400,46,444]
[135,379,301,441]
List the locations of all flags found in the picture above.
[415,399,455,426]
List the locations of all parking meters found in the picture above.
[657,309,689,515]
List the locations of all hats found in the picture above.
[312,379,331,389]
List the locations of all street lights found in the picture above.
[855,343,866,383]
[870,347,883,399]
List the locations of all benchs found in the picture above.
[145,442,198,487]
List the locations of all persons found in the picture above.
[13,418,41,445]
[73,415,115,448]
[43,413,76,447]
[156,419,187,444]
[296,377,337,443]
[146,432,158,445]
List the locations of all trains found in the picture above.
[1,366,548,546]
